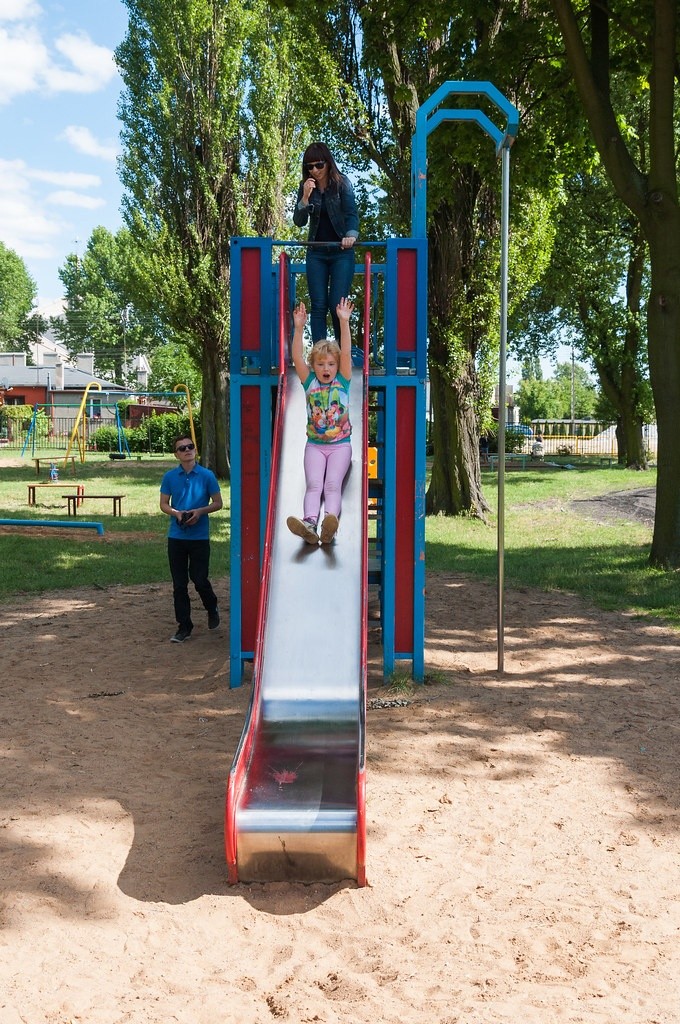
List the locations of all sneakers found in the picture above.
[208,606,220,630]
[170,625,191,642]
[286,516,319,545]
[319,514,338,543]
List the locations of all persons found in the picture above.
[159,436,221,647]
[294,142,361,345]
[287,297,355,546]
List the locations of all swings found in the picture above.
[145,393,166,457]
[105,392,129,459]
[52,405,70,450]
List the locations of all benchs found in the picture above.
[481,452,517,463]
[109,453,143,461]
[32,456,77,475]
[489,455,527,470]
[62,492,125,518]
[26,482,87,508]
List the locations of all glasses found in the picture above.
[176,444,195,452]
[305,161,327,170]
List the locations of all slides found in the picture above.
[231,365,375,883]
